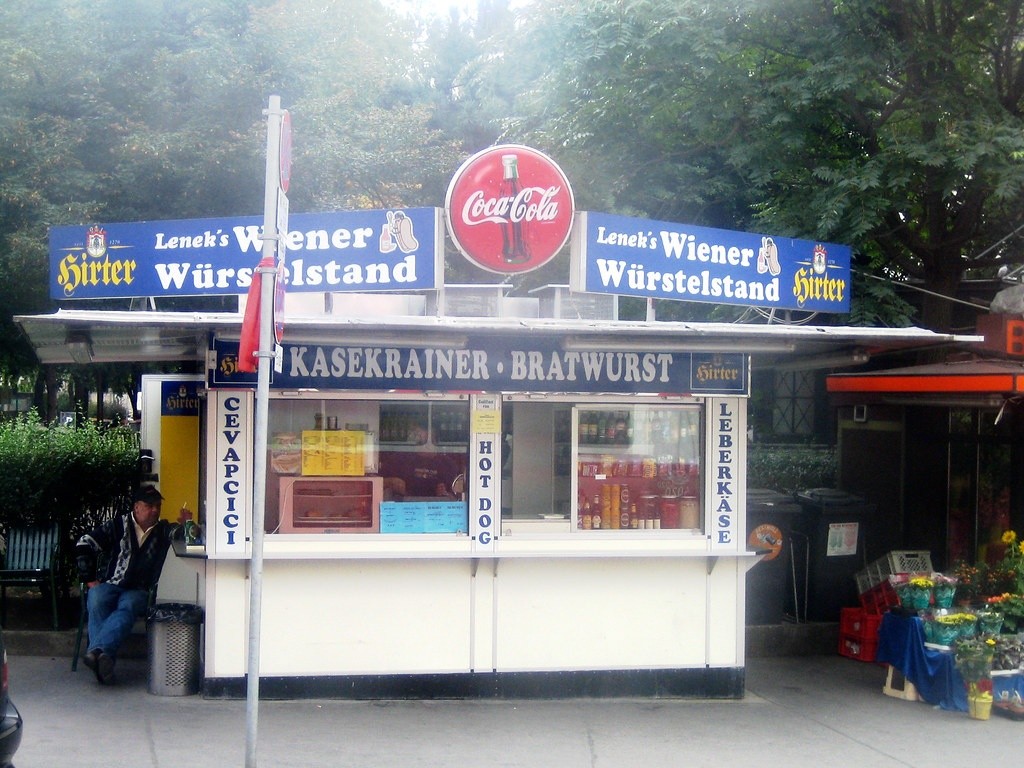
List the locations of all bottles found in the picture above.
[630,491,698,529]
[380,405,428,441]
[433,405,468,442]
[578,409,699,445]
[579,489,601,529]
[186,520,195,545]
[500,155,532,263]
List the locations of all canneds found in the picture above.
[600,482,631,529]
[184,519,197,546]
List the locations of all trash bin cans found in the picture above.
[795,488,864,622]
[144,602,206,697]
[746,487,802,622]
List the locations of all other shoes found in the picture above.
[83,650,117,686]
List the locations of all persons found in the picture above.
[379,415,467,497]
[75,485,193,684]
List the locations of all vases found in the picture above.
[967,694,994,720]
[932,620,960,648]
[923,619,936,643]
[910,585,931,610]
[960,621,977,638]
[932,584,957,609]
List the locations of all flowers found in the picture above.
[907,530,1024,698]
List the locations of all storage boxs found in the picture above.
[854,550,933,595]
[838,606,881,640]
[838,633,879,662]
[859,572,930,616]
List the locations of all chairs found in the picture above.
[70,551,157,673]
[0,519,63,631]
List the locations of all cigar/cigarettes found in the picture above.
[183,502,186,509]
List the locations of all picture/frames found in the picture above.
[979,621,1004,637]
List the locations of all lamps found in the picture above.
[64,328,93,364]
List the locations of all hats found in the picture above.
[133,484,166,503]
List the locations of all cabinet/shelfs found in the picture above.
[881,612,1024,715]
